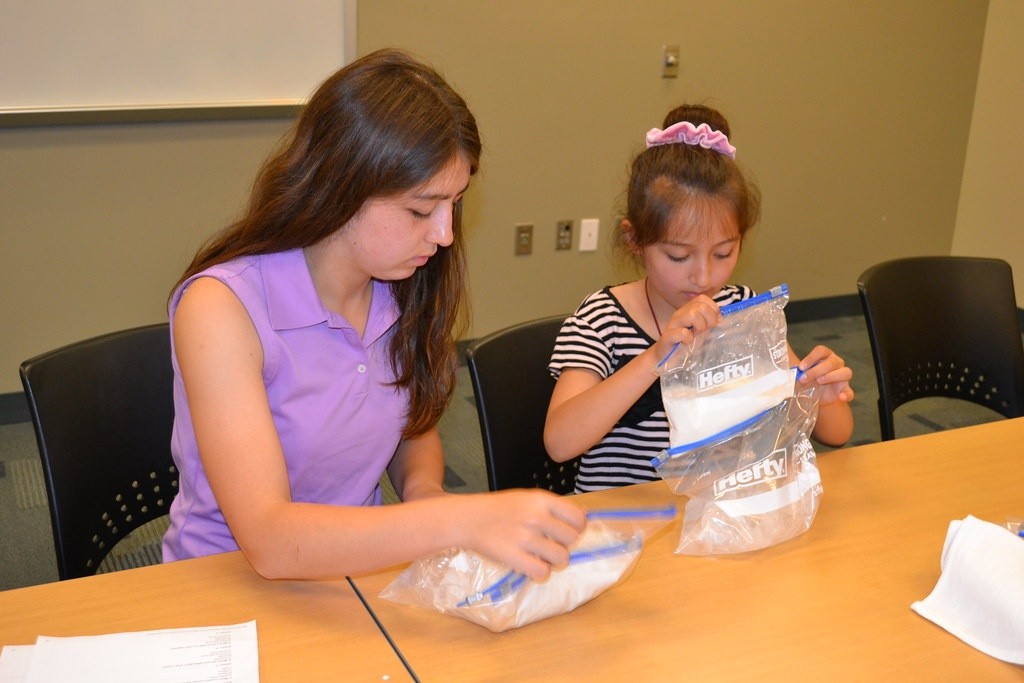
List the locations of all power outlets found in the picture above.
[556,219,573,250]
[660,45,679,78]
[515,224,533,255]
[578,218,599,251]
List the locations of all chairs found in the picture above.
[857,255,1024,443]
[465,313,581,495]
[20,321,180,582]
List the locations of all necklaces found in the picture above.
[644,277,664,338]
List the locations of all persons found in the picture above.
[541,102,857,495]
[159,45,590,584]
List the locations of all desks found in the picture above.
[0,415,1024,682]
